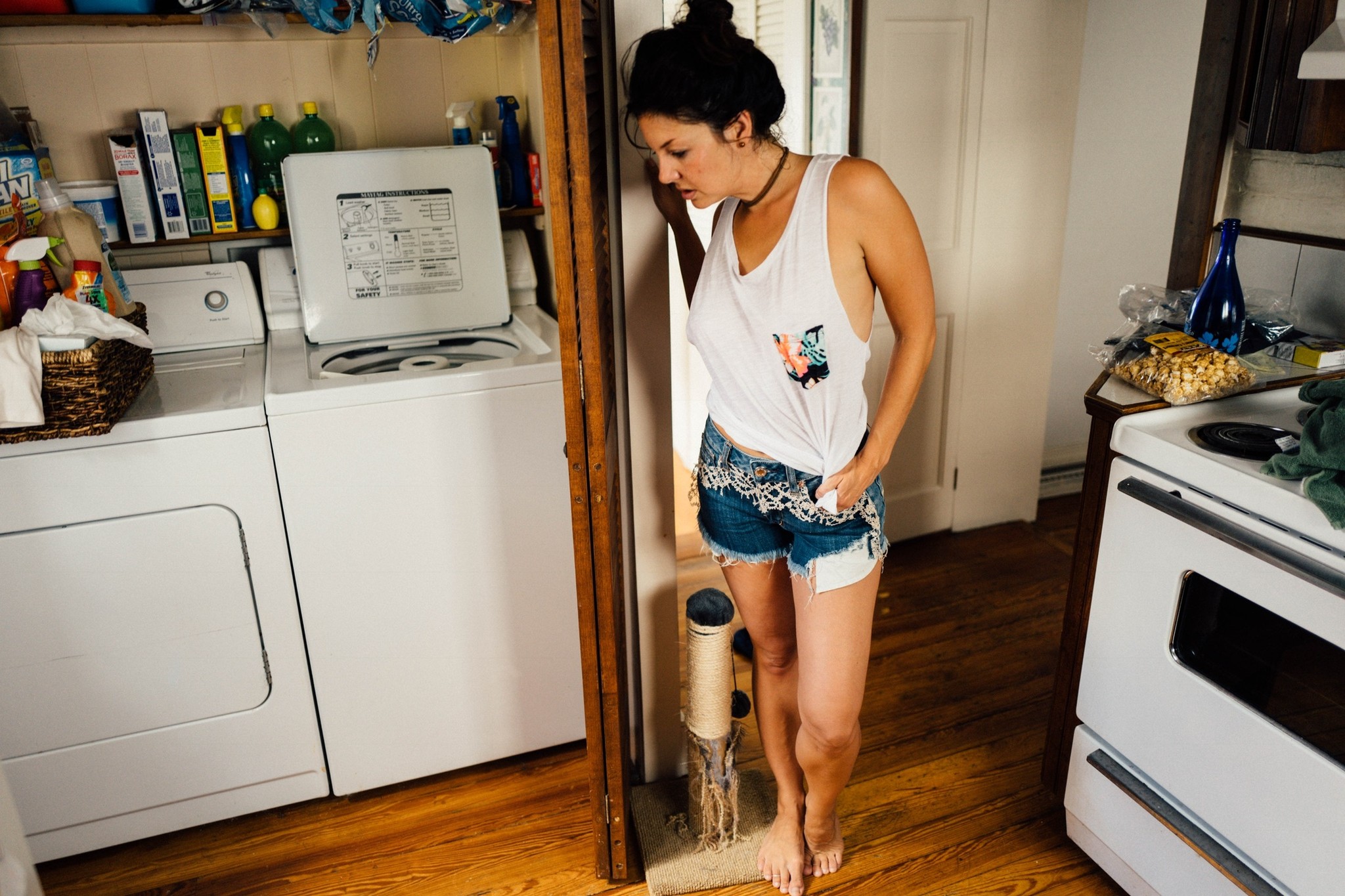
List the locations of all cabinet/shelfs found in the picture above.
[0,8,415,249]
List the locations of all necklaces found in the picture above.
[741,146,789,209]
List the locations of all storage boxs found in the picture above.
[137,108,191,240]
[109,126,158,243]
[174,128,212,235]
[192,120,237,236]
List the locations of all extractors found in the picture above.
[1296,1,1344,81]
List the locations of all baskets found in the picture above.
[0,299,156,447]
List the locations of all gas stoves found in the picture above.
[1110,386,1345,555]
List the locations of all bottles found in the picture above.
[293,102,335,153]
[250,104,293,231]
[477,129,514,213]
[1185,218,1247,357]
[34,178,137,316]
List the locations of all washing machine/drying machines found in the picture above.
[249,143,584,798]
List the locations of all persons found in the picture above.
[618,0,938,896]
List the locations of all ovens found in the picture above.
[1063,455,1344,895]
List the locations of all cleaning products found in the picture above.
[494,93,530,205]
[223,103,255,229]
[446,101,480,144]
